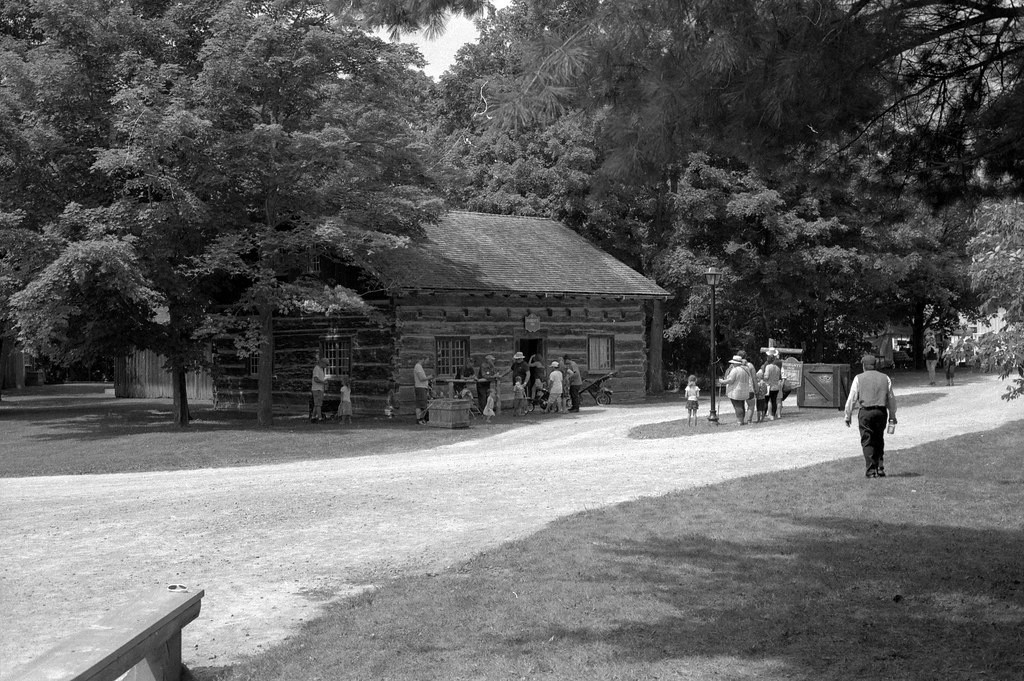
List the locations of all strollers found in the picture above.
[526,387,559,413]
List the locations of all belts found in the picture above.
[860,406,885,410]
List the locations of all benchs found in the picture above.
[308,399,351,424]
[1,583,205,681]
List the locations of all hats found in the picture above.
[728,355,743,365]
[486,355,496,360]
[688,375,697,383]
[321,358,331,366]
[547,361,559,368]
[513,351,524,359]
[766,347,779,356]
[861,355,876,365]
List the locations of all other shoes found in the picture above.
[770,415,774,421]
[877,471,886,476]
[737,422,744,425]
[319,419,327,424]
[310,418,317,424]
[748,420,753,424]
[762,418,766,422]
[867,473,876,478]
[755,419,760,423]
[777,415,781,419]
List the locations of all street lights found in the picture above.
[705,267,723,425]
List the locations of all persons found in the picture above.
[312,358,329,422]
[923,327,938,385]
[685,375,701,427]
[941,344,955,386]
[845,356,897,478]
[415,352,582,424]
[338,374,353,423]
[719,347,784,425]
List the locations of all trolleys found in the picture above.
[566,371,620,407]
